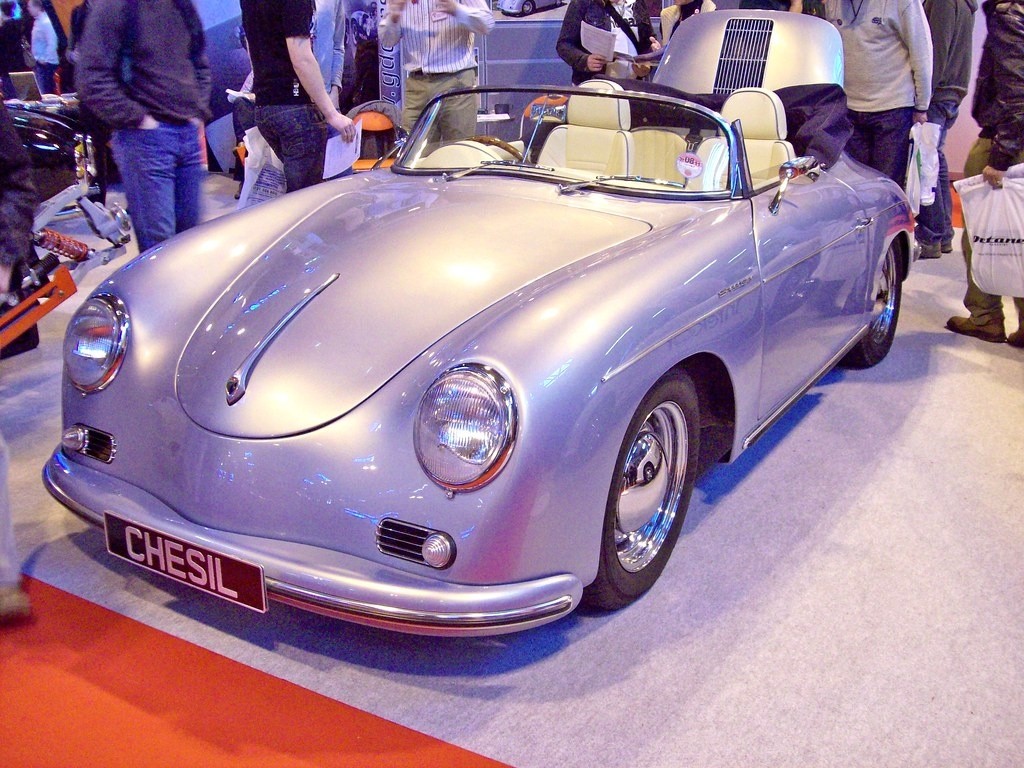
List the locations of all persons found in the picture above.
[915,0,978,259]
[0,0,82,101]
[73,0,213,252]
[379,0,495,160]
[947,0,1024,347]
[312,0,353,181]
[232,70,256,200]
[240,0,357,192]
[0,95,37,309]
[556,0,933,196]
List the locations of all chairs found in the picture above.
[353,111,397,174]
[687,87,796,191]
[519,94,568,139]
[536,79,633,177]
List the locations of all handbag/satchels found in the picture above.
[238,126,289,212]
[951,162,1024,298]
[903,123,940,218]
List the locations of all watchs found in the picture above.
[0,291,19,307]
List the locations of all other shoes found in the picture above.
[918,240,942,258]
[942,241,953,253]
[947,316,1006,342]
[1007,325,1024,348]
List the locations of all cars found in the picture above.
[0,89,113,215]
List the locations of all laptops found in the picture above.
[9,72,42,101]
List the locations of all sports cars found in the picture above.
[39,7,921,638]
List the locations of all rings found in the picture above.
[997,181,1000,184]
